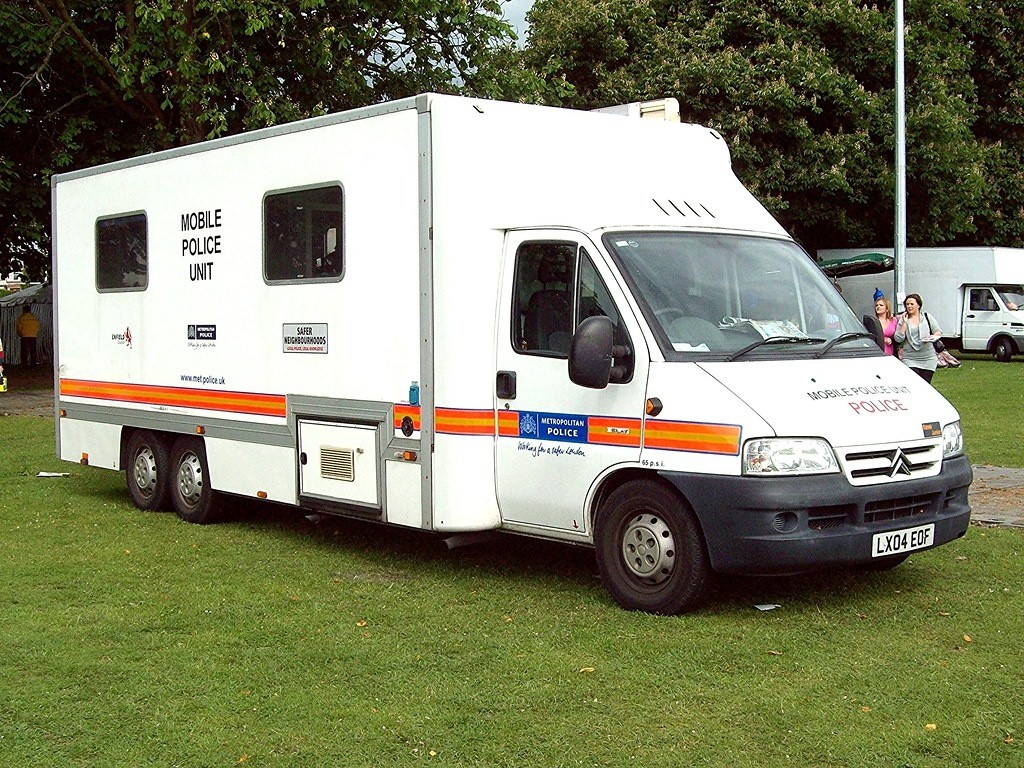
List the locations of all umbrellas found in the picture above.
[816,253,895,283]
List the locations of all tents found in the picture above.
[0,283,53,365]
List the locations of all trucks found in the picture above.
[49,94,973,614]
[816,247,1024,361]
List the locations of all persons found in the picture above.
[894,293,943,385]
[874,297,898,356]
[16,305,40,372]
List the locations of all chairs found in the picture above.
[642,249,724,336]
[524,247,593,351]
